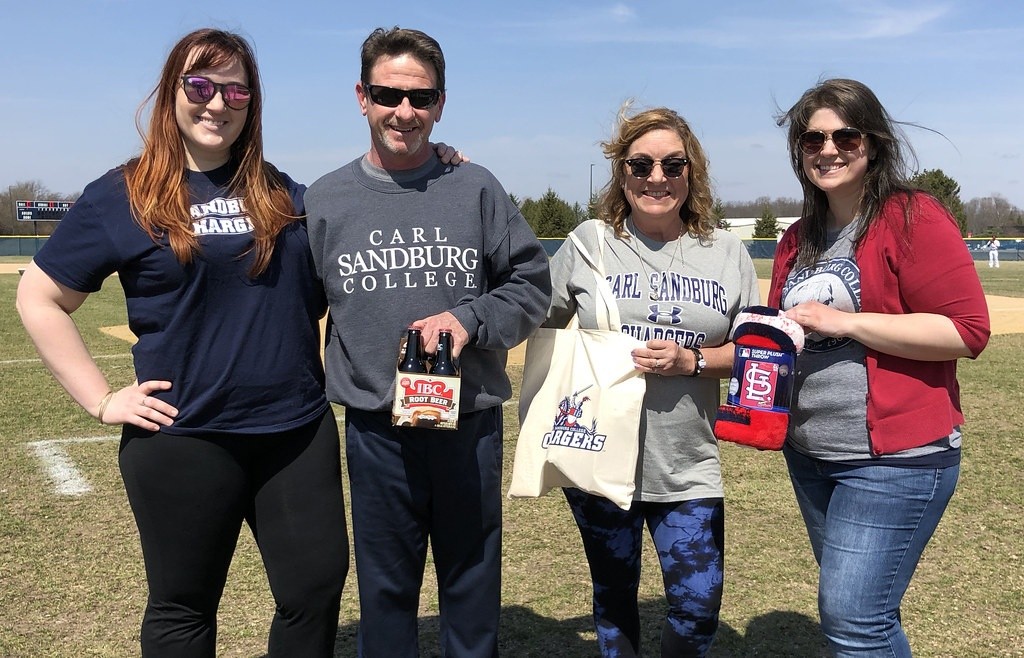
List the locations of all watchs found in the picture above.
[686,346,706,379]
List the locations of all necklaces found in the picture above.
[627,210,684,301]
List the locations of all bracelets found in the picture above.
[97,389,113,423]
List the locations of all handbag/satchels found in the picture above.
[507,218,650,512]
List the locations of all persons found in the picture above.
[532,105,766,658]
[298,27,553,658]
[766,76,990,658]
[9,27,472,658]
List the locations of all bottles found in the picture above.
[429,328,457,376]
[397,327,428,373]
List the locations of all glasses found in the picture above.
[175,75,252,110]
[797,126,871,153]
[622,157,689,179]
[361,81,446,110]
